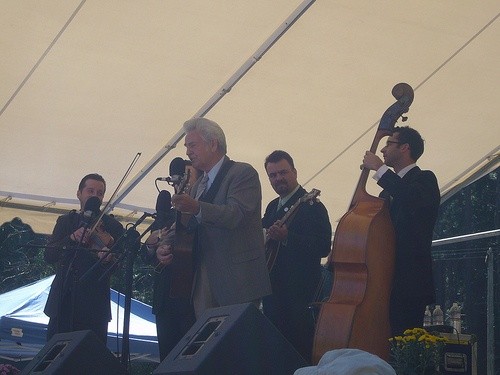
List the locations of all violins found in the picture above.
[77,215,114,257]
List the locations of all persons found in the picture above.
[150,157,198,363]
[155,118,273,320]
[360,126,441,375]
[262,150,332,364]
[43,173,125,348]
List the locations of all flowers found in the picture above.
[386,328,447,375]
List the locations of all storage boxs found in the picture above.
[437,333,477,375]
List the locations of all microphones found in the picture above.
[84,197,102,229]
[155,190,172,241]
[157,175,182,186]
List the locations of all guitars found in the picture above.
[263,189,322,276]
[164,165,196,301]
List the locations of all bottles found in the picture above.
[449,303,461,335]
[423,306,431,328]
[432,305,444,326]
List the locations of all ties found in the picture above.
[192,175,209,224]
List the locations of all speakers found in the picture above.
[16,329,133,375]
[150,302,314,375]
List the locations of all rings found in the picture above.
[173,206,176,209]
[174,203,176,206]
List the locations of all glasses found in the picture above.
[386,141,401,147]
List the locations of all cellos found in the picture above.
[311,82,414,364]
